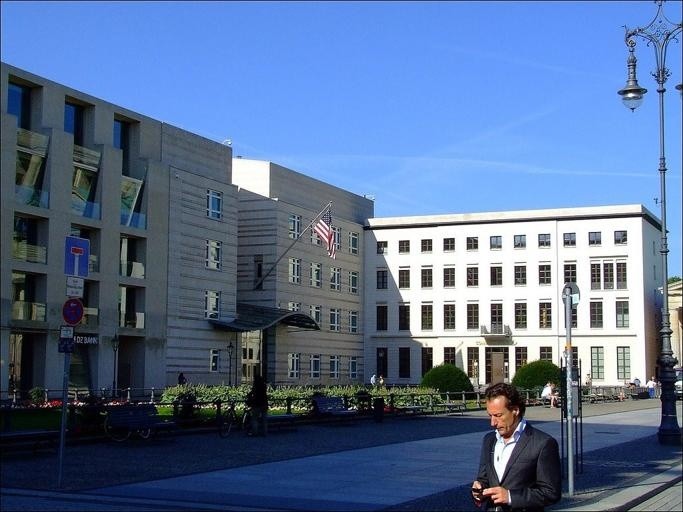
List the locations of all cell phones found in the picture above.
[472,488,485,493]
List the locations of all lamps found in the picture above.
[618,25,648,115]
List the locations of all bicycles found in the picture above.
[105,415,150,441]
[219,398,257,439]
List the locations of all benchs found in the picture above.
[311,397,359,424]
[437,403,466,415]
[102,403,177,442]
[537,392,632,407]
[395,406,427,419]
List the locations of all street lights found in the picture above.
[617,1,683,445]
[226,341,235,388]
[110,334,121,397]
[10,331,20,403]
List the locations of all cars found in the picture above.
[673,368,683,400]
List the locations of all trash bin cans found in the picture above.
[374,398,384,422]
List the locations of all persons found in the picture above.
[629,376,658,399]
[541,381,559,408]
[371,375,378,386]
[246,377,268,437]
[178,373,187,385]
[379,374,384,386]
[471,383,561,512]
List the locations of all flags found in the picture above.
[313,210,336,260]
